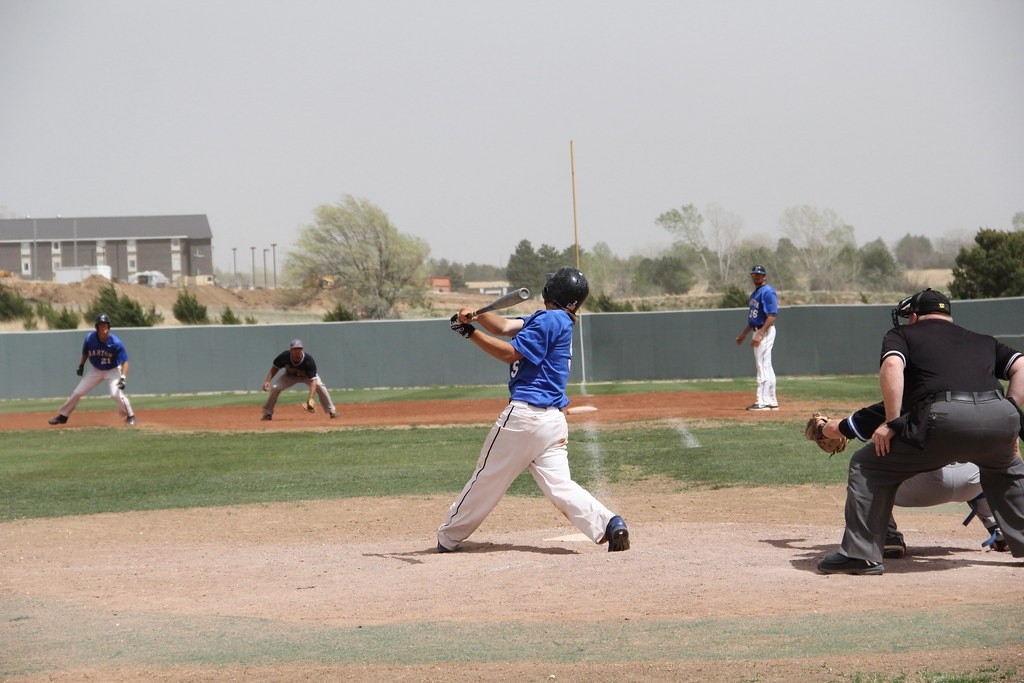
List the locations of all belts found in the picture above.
[951,461,967,465]
[922,389,1005,403]
[753,324,775,331]
[508,399,562,412]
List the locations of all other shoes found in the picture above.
[48,414,68,424]
[127,416,134,425]
[328,408,337,418]
[261,413,272,421]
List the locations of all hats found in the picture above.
[750,265,765,275]
[290,339,303,349]
[898,288,951,317]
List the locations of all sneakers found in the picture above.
[991,537,1009,550]
[606,517,631,552]
[436,541,452,552]
[746,403,779,410]
[883,537,906,557]
[817,552,884,575]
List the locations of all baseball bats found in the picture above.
[458,286,531,319]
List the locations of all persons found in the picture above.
[437,267,631,554]
[736,265,778,410]
[48,315,135,425]
[805,289,1024,574]
[261,339,338,421]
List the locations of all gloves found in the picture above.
[450,313,477,339]
[117,378,126,389]
[75,365,85,376]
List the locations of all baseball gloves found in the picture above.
[301,398,316,413]
[802,413,849,453]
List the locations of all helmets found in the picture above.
[542,265,589,314]
[95,313,110,330]
